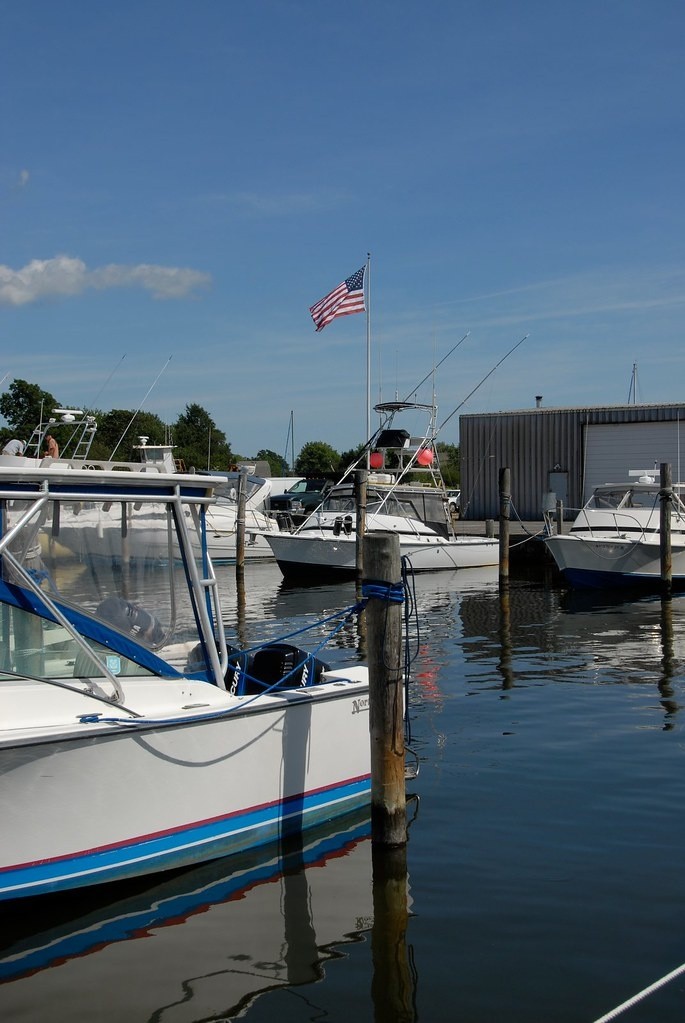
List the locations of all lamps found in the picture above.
[417,446,434,466]
[369,449,384,469]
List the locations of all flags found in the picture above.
[310,264,367,333]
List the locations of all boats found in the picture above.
[244,330,501,572]
[0,380,415,901]
[543,458,685,582]
[0,792,423,1023]
[0,351,304,559]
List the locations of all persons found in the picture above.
[2,439,27,458]
[41,434,59,459]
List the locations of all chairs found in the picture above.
[72,645,129,678]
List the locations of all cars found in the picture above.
[442,489,460,513]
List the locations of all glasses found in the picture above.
[45,435,48,437]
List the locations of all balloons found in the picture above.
[418,447,432,465]
[370,449,383,469]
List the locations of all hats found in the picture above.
[23,440,27,448]
